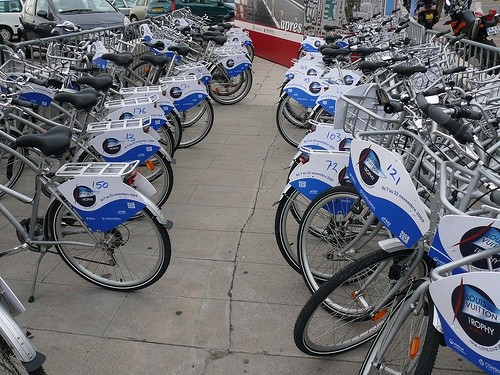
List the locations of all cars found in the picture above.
[19,0,131,54]
[108,0,236,24]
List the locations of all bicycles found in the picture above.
[0,10,260,375]
[275,8,500,374]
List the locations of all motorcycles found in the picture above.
[442,0,500,69]
[403,0,440,28]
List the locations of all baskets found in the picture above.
[0,41,86,81]
[356,13,500,139]
[431,162,500,257]
[431,245,500,375]
[333,83,399,149]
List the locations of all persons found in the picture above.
[410,0,418,20]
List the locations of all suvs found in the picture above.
[0,0,27,41]
